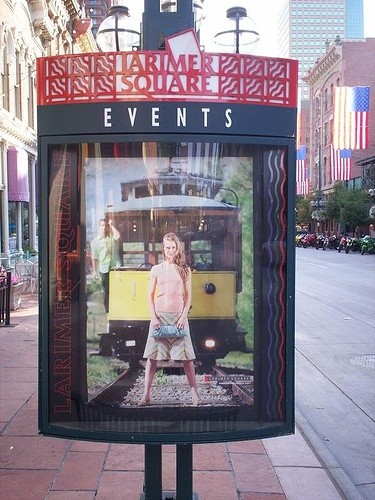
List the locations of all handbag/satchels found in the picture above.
[153,324,186,339]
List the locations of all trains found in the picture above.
[90,159,248,369]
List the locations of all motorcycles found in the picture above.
[338,235,352,253]
[322,235,338,251]
[297,234,307,248]
[345,235,371,254]
[295,233,300,247]
[316,234,326,250]
[361,238,375,255]
[302,234,315,248]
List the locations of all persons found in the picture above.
[89,218,125,314]
[144,233,199,404]
